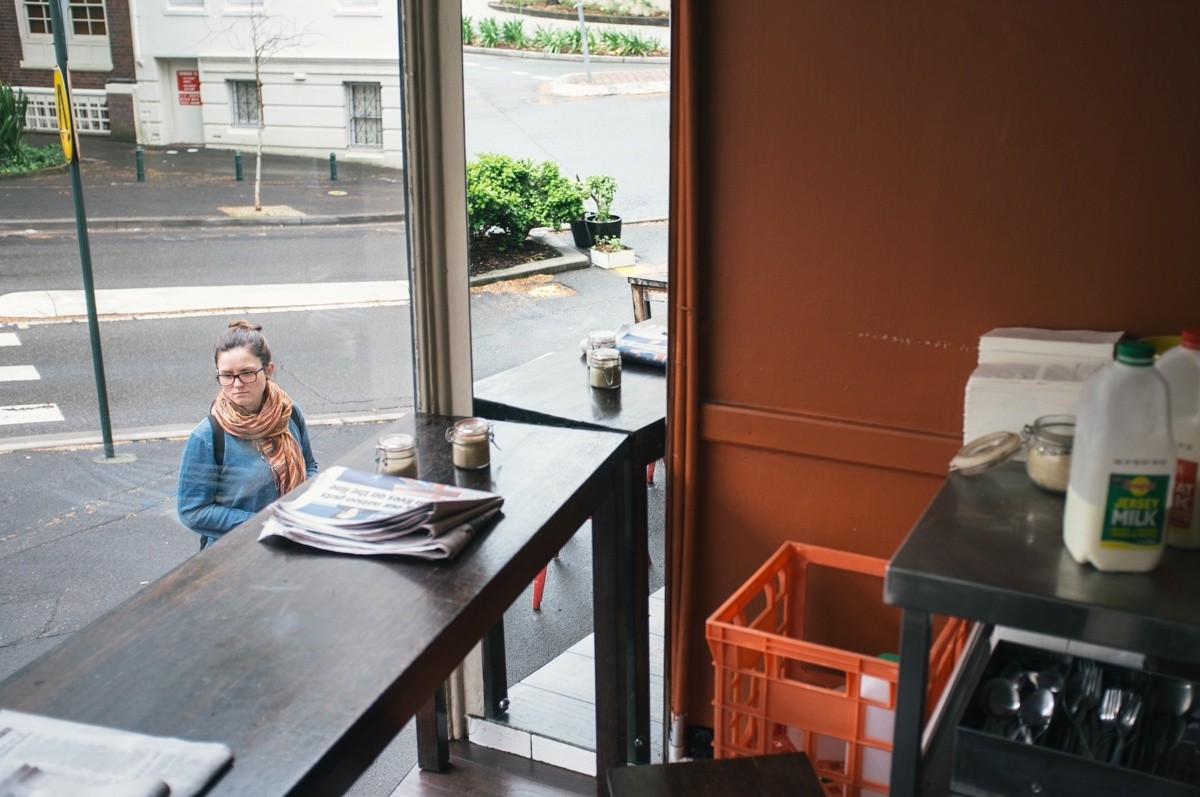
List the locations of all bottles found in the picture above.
[769,652,901,797]
[1062,327,1200,574]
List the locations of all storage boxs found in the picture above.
[711,539,970,797]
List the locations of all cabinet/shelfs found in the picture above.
[882,440,1200,797]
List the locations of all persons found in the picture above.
[177,321,317,550]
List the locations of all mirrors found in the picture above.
[462,0,672,767]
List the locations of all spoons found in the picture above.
[978,670,1064,748]
[1112,679,1194,780]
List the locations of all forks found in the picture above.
[1059,651,1122,761]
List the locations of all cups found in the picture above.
[445,418,495,470]
[375,433,419,479]
[586,330,621,388]
[1022,412,1075,494]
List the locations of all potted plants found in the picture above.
[587,235,636,270]
[576,171,622,247]
[546,173,594,248]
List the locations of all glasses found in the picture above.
[216,363,268,386]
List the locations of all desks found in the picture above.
[0,414,653,797]
[474,320,666,465]
[626,264,668,324]
